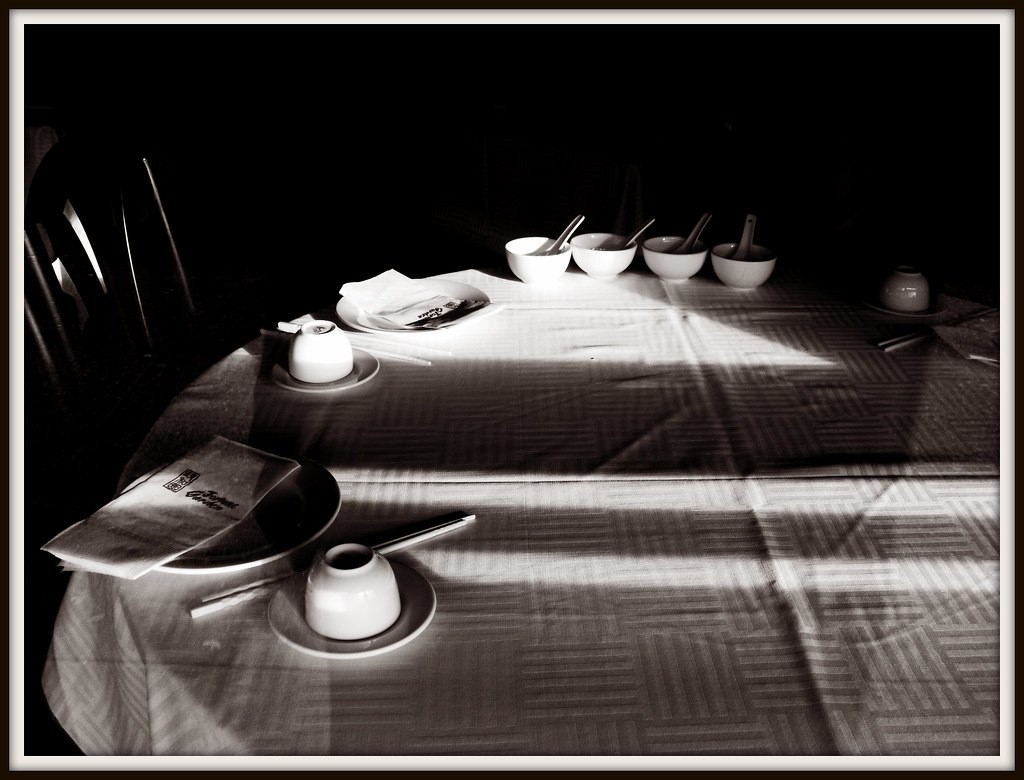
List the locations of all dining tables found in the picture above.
[46,268,1001,757]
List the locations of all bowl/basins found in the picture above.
[711,243,777,291]
[506,237,572,284]
[570,233,637,280]
[305,543,401,642]
[878,268,931,312]
[642,237,709,282]
[289,321,353,382]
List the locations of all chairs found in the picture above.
[24,120,240,510]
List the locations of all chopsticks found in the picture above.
[878,330,932,353]
[259,321,452,367]
[190,514,476,617]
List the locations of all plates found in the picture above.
[118,456,342,578]
[267,560,436,658]
[866,288,952,318]
[269,348,379,393]
[336,278,491,334]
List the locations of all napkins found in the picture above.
[339,268,504,330]
[930,325,1000,363]
[40,434,301,580]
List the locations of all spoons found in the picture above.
[535,215,584,256]
[671,215,712,255]
[592,218,655,251]
[733,215,756,260]
